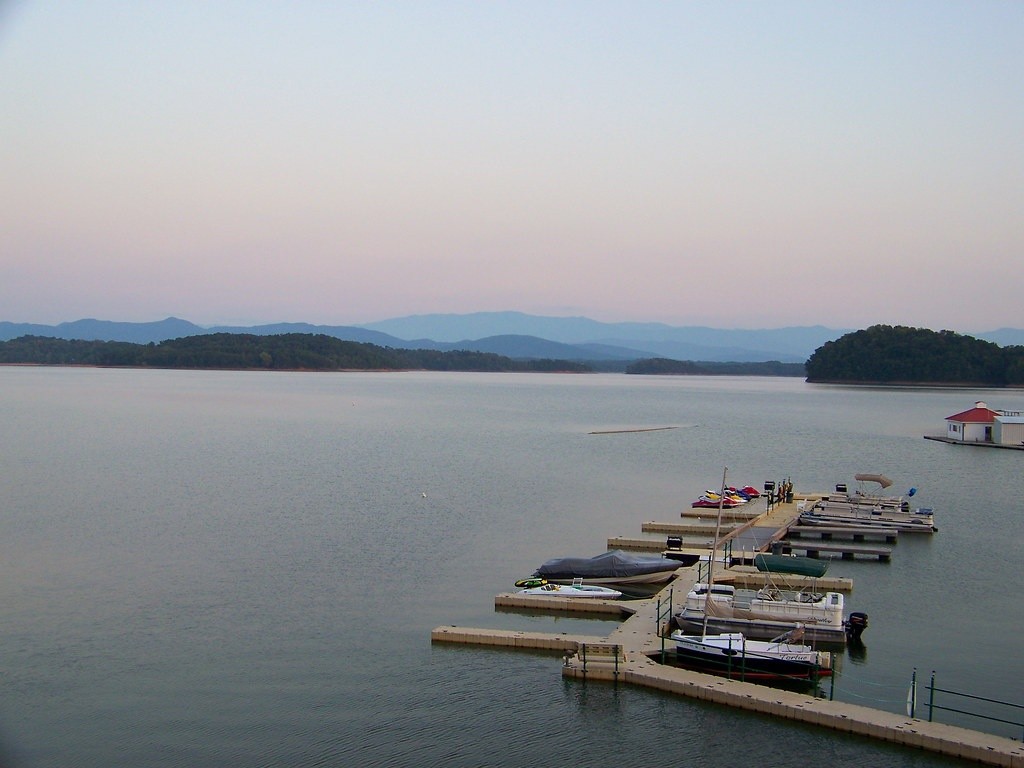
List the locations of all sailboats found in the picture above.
[670,466,832,694]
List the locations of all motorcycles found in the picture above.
[691,484,760,509]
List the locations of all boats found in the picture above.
[673,583,869,652]
[530,549,684,584]
[516,583,622,600]
